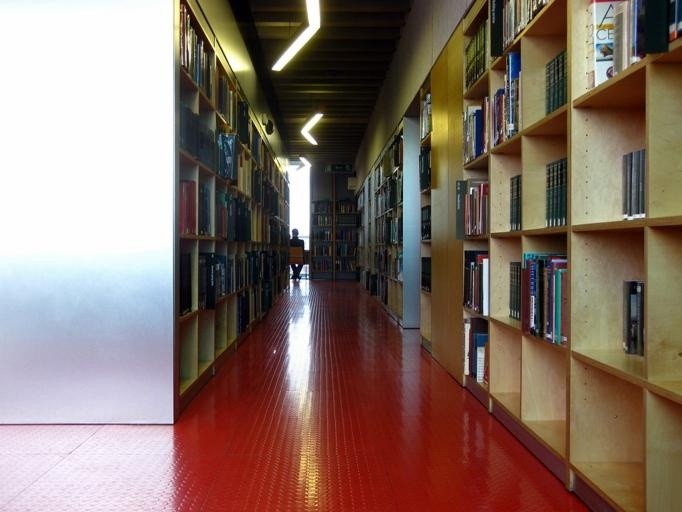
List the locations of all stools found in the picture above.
[289,247,310,280]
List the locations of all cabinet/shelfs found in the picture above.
[463,0,682,512]
[311,155,359,281]
[173,0,290,419]
[356,71,462,354]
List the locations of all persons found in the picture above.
[290,230,305,280]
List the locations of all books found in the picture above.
[509,157,568,345]
[463,0,682,164]
[455,178,489,387]
[622,280,644,356]
[622,150,647,221]
[178,3,291,334]
[311,88,432,319]
